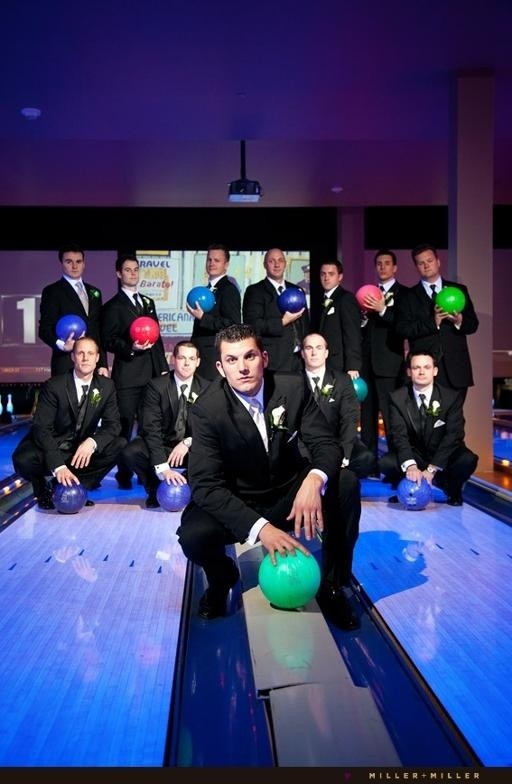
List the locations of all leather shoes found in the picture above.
[38,481,54,510]
[368,467,380,481]
[318,582,356,629]
[86,499,94,506]
[117,477,132,489]
[146,489,158,508]
[197,565,240,619]
[389,495,398,504]
[446,484,463,505]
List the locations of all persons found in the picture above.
[298,265,310,294]
[104,254,171,489]
[395,244,479,407]
[191,245,241,381]
[12,337,127,510]
[387,352,478,506]
[292,332,376,477]
[427,466,436,475]
[176,324,361,631]
[38,247,103,378]
[120,340,212,508]
[359,251,411,476]
[309,260,363,381]
[241,247,308,429]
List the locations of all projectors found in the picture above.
[225,174,263,204]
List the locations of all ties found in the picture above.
[132,294,143,315]
[206,282,211,290]
[278,286,297,350]
[76,282,88,316]
[78,386,88,432]
[419,395,428,435]
[312,377,322,400]
[322,294,328,301]
[251,403,268,451]
[379,286,384,293]
[429,284,438,298]
[175,384,188,439]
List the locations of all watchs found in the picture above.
[182,437,191,449]
